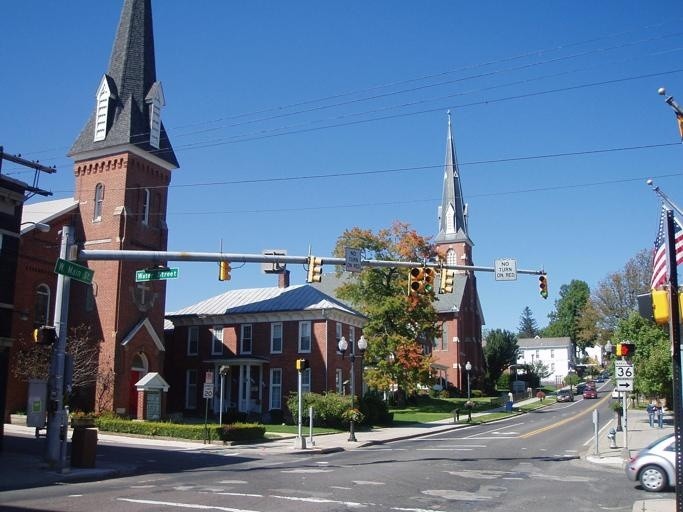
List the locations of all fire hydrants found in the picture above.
[608,428,617,450]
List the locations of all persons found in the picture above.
[652,396,664,428]
[527,386,533,398]
[508,391,514,402]
[647,399,655,427]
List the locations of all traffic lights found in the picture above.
[296,359,309,371]
[636,289,671,324]
[619,343,636,358]
[539,276,548,299]
[410,267,435,294]
[33,325,58,347]
[218,261,231,281]
[440,268,455,295]
[307,256,323,282]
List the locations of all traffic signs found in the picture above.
[53,258,94,286]
[135,266,179,283]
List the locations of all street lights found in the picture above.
[464,361,471,400]
[337,335,367,441]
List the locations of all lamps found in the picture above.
[20,222,51,233]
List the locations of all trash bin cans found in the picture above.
[70,417,97,467]
[506,402,512,413]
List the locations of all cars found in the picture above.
[613,387,624,399]
[626,434,675,491]
[556,389,574,402]
[592,372,612,382]
[575,381,598,400]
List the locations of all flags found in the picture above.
[651,201,683,291]
